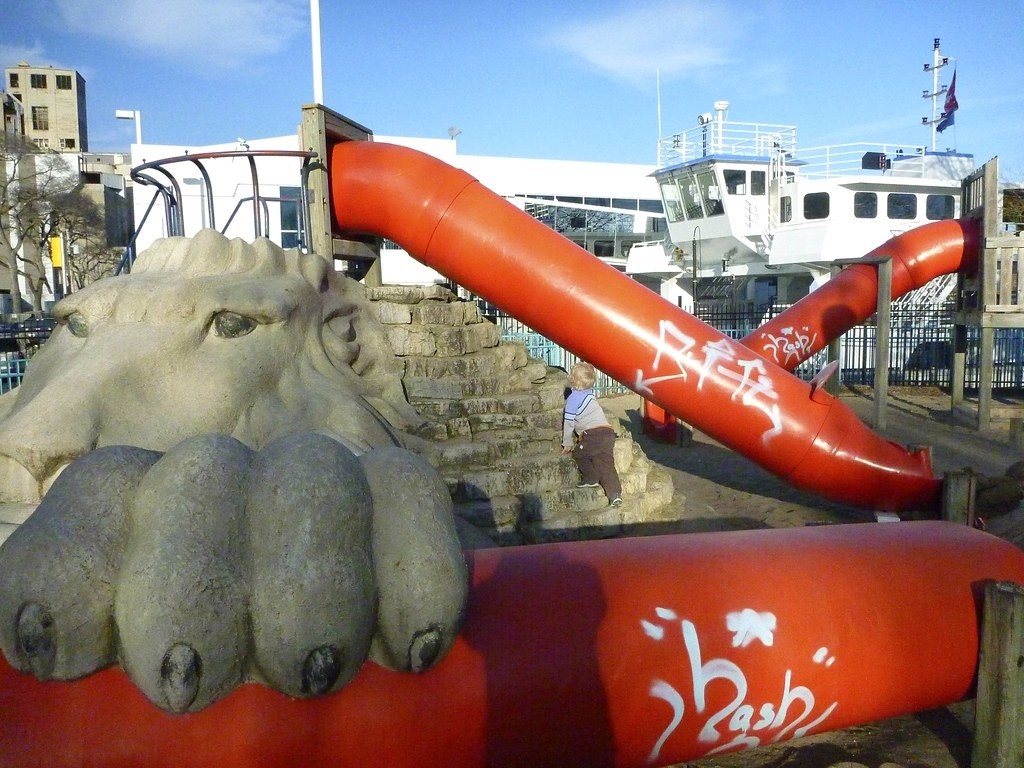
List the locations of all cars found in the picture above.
[0,293,57,349]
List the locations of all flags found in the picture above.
[937,68,959,131]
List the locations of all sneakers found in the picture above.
[576,480,599,488]
[608,493,622,508]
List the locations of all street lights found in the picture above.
[115,109,141,145]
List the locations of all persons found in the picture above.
[560,363,621,508]
